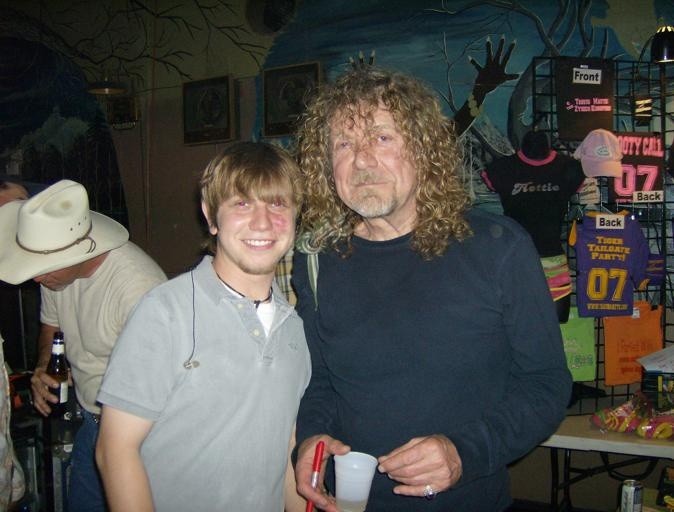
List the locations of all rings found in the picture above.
[425,483,437,501]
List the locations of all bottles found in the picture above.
[44,330,69,417]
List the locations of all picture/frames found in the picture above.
[181,73,236,146]
[262,60,324,138]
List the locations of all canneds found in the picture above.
[617,479,644,512]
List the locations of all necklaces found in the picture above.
[215,273,275,309]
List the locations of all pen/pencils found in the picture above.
[306,441,325,512]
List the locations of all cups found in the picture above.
[332,450,380,512]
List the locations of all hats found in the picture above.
[575,129,624,178]
[0,179,129,285]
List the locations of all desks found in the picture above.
[539,414,673,512]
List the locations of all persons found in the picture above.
[481,135,585,325]
[1,179,169,512]
[290,61,574,511]
[1,334,26,512]
[94,142,313,511]
[349,34,520,139]
